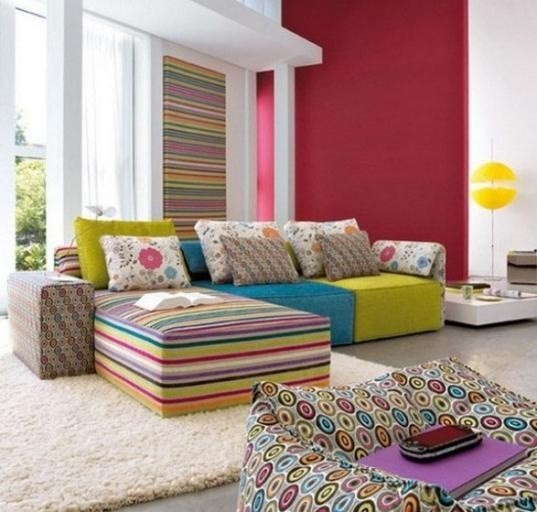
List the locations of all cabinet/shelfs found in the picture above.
[506,250,537,285]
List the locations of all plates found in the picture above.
[476,295,501,301]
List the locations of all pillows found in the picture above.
[316,230,380,282]
[99,235,192,291]
[194,220,285,285]
[219,234,302,287]
[73,217,176,290]
[284,218,360,279]
[371,239,442,277]
[181,241,209,280]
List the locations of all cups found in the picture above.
[460,285,473,300]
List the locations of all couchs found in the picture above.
[192,239,446,346]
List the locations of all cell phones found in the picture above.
[400,420,483,462]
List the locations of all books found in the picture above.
[352,422,527,499]
[132,290,223,311]
[482,286,537,299]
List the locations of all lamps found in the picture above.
[471,138,518,288]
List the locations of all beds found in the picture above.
[5,269,333,417]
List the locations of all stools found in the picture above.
[237,353,537,512]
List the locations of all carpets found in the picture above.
[0,316,402,511]
